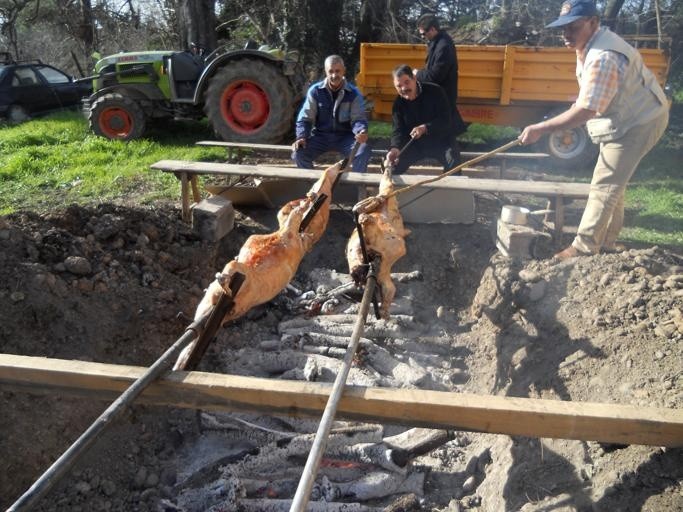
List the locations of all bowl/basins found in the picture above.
[499,205,531,225]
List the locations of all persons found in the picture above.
[288,54,372,173]
[409,12,458,112]
[516,1,672,261]
[381,65,467,177]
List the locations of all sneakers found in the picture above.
[551,243,615,265]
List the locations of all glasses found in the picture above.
[419,31,426,36]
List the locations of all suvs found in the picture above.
[0,57,92,130]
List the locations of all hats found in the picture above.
[544,0,596,28]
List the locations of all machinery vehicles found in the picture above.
[71,32,310,152]
